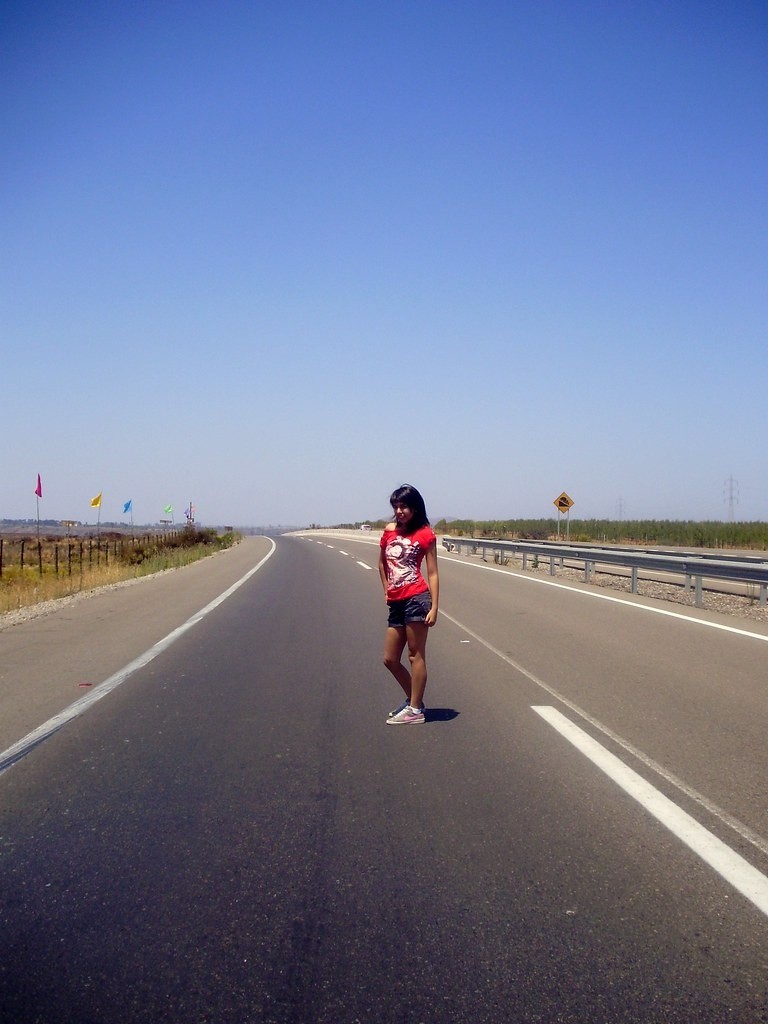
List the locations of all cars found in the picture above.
[360,525,372,531]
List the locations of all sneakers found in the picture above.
[386,697,426,726]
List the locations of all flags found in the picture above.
[90,490,102,508]
[184,506,191,516]
[34,473,42,497]
[123,499,132,513]
[164,504,172,513]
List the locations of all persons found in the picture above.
[378,483,440,726]
